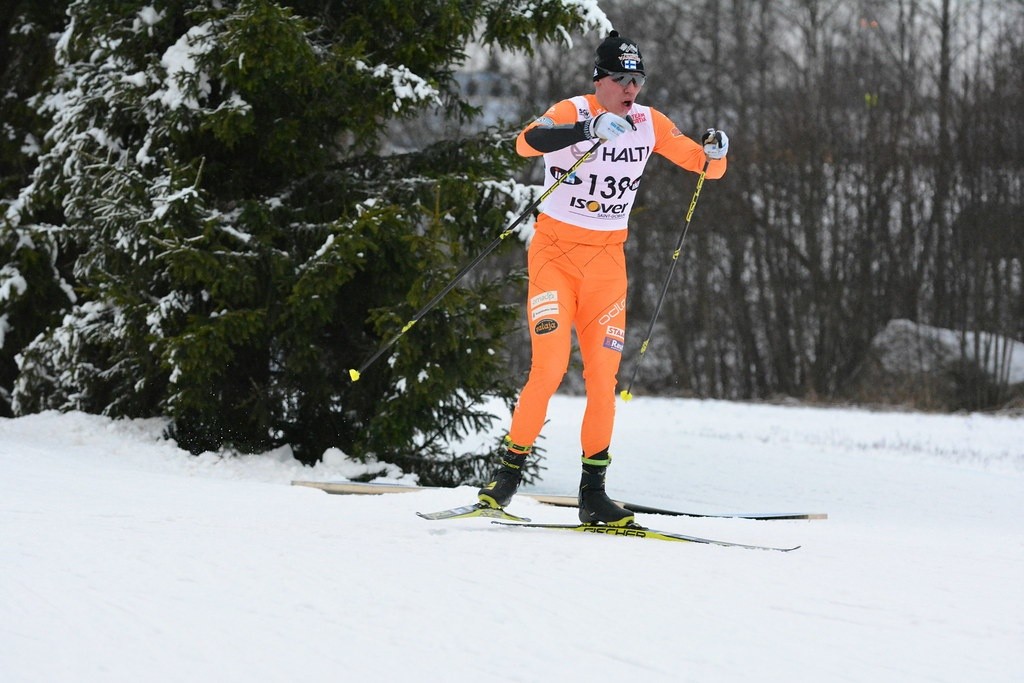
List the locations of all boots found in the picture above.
[578,457,636,526]
[477,450,525,509]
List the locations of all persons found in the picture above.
[476,29,729,526]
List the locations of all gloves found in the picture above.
[703,130,729,159]
[589,111,633,141]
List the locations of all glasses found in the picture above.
[602,69,646,87]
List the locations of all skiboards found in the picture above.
[413,491,803,555]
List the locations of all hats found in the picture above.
[592,30,645,81]
